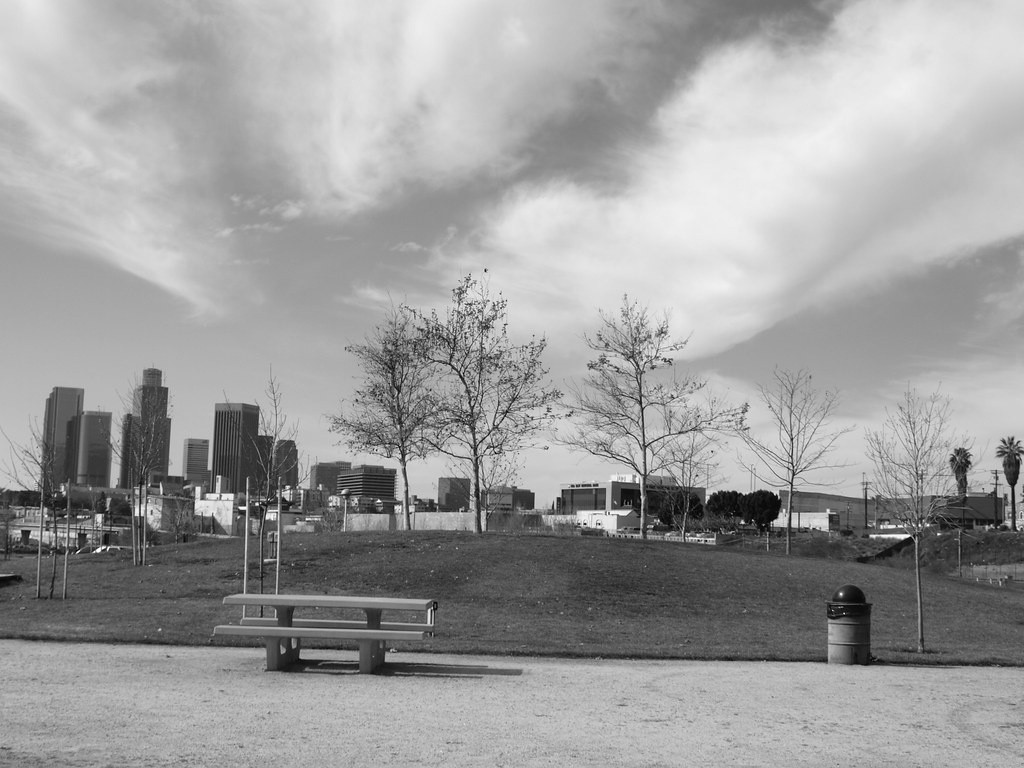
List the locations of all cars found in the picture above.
[76,544,129,554]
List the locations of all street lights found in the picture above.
[340,489,352,532]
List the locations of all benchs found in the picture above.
[214,625,424,641]
[239,618,435,632]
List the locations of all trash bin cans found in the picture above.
[826,584,873,665]
[78,533,87,546]
[21,530,31,545]
[267,530,278,559]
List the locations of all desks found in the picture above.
[223,593,438,674]
